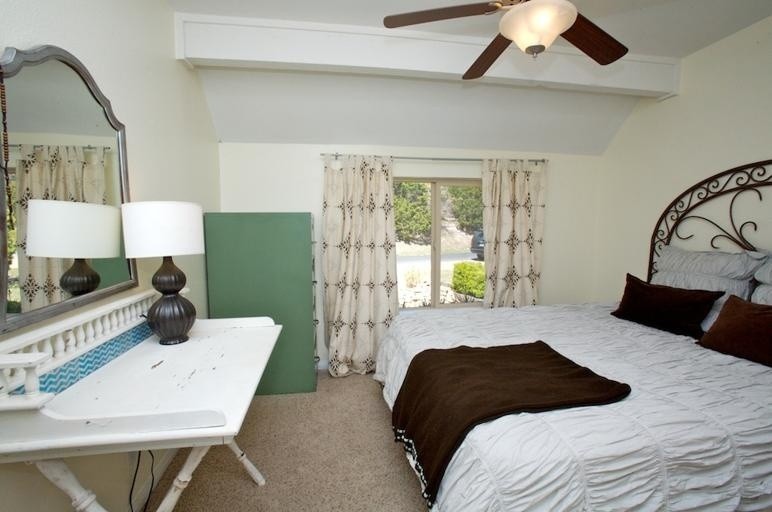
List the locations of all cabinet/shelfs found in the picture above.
[204,212,319,396]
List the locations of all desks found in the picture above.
[0,285,283,512]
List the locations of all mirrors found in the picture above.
[0,45,139,339]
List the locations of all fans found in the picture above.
[383,0,629,81]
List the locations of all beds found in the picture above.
[373,157,772,512]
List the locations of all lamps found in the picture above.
[26,198,122,301]
[122,202,204,347]
[498,0,579,60]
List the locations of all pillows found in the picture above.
[609,271,727,341]
[650,245,768,332]
[693,294,772,368]
[751,255,772,305]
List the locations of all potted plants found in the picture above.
[453,261,486,302]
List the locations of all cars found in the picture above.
[470,229,485,259]
